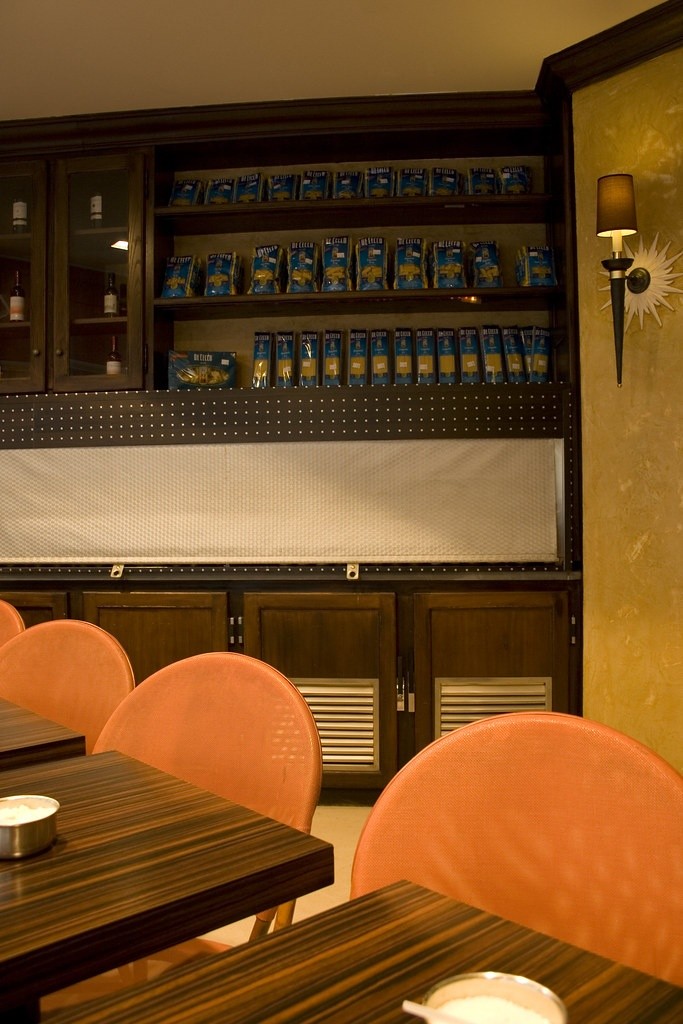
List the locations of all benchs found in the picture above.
[91,650,324,948]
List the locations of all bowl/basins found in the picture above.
[0,795,61,859]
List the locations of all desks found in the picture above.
[1,750,334,1015]
[0,698,87,772]
[45,879,682,1023]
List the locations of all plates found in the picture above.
[422,970,568,1024]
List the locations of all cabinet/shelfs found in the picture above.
[0,90,584,808]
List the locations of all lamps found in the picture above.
[596,172,683,388]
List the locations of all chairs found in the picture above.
[0,619,135,754]
[350,710,683,993]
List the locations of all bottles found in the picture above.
[107,336,122,374]
[12,180,28,233]
[9,269,25,320]
[103,272,119,318]
[90,176,104,229]
